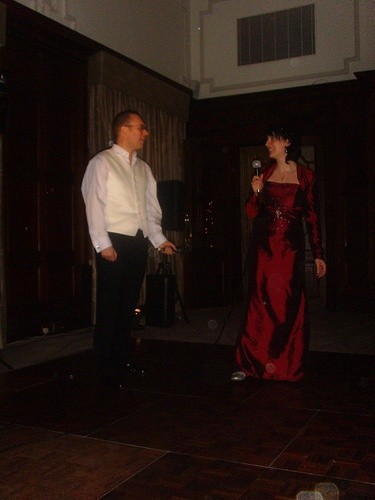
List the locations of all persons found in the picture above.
[81,110,176,390]
[231,125,327,386]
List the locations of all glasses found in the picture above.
[122,124,147,131]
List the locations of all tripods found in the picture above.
[134,261,188,325]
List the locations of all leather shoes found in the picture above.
[121,363,146,374]
[101,373,125,389]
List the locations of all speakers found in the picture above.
[156,181,185,230]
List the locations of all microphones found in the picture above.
[253,160,263,193]
[158,248,187,254]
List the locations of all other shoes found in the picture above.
[230,371,247,380]
